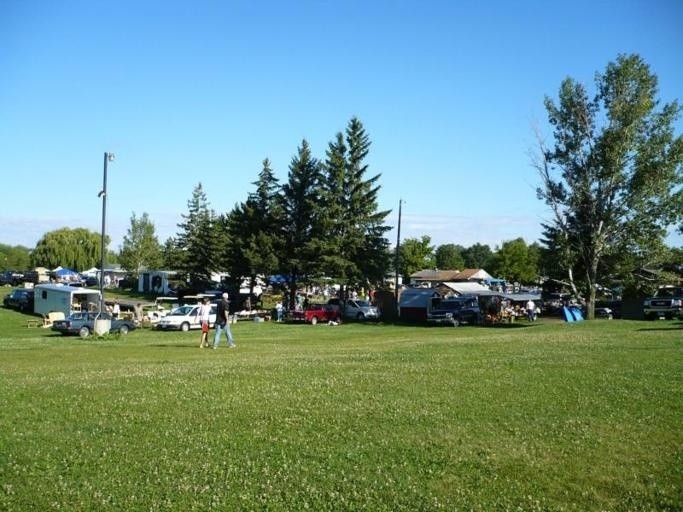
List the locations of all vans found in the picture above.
[328,297,379,320]
[161,301,222,331]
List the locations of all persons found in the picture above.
[134,302,142,329]
[324,316,344,325]
[351,289,357,298]
[111,297,120,317]
[243,297,309,323]
[482,295,536,325]
[211,292,236,349]
[197,297,216,348]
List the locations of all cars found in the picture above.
[51,312,137,338]
[0,269,23,287]
[2,288,35,314]
[506,285,542,294]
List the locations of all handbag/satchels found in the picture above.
[202,322,209,333]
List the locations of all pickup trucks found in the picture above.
[288,302,337,325]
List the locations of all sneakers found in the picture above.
[199,343,236,349]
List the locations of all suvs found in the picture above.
[643,285,683,321]
[427,294,480,327]
[60,279,84,288]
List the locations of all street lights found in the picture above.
[99,150,115,312]
[394,197,409,303]
